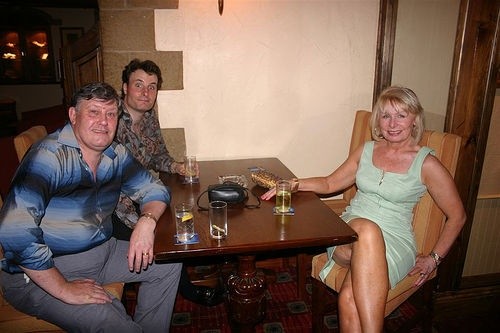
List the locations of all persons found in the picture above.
[0,81,182,333]
[261,87,466,333]
[111,58,217,302]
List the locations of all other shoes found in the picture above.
[184,284,220,305]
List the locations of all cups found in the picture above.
[174,203,194,242]
[276,180,291,212]
[274,216,291,241]
[209,201,227,240]
[183,156,197,183]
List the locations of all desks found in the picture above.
[144,155,362,333]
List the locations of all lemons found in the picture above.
[181,214,193,223]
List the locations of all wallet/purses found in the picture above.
[252,171,284,190]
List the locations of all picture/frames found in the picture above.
[60,26,85,48]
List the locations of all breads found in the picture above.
[251,169,299,193]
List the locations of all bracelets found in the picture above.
[290,177,299,194]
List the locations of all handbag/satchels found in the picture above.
[197,181,261,210]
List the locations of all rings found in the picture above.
[420,273,426,277]
[142,253,149,255]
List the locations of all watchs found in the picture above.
[429,251,445,263]
[139,213,158,223]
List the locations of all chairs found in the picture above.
[13,125,48,164]
[0,192,126,333]
[281,109,381,299]
[311,129,463,333]
